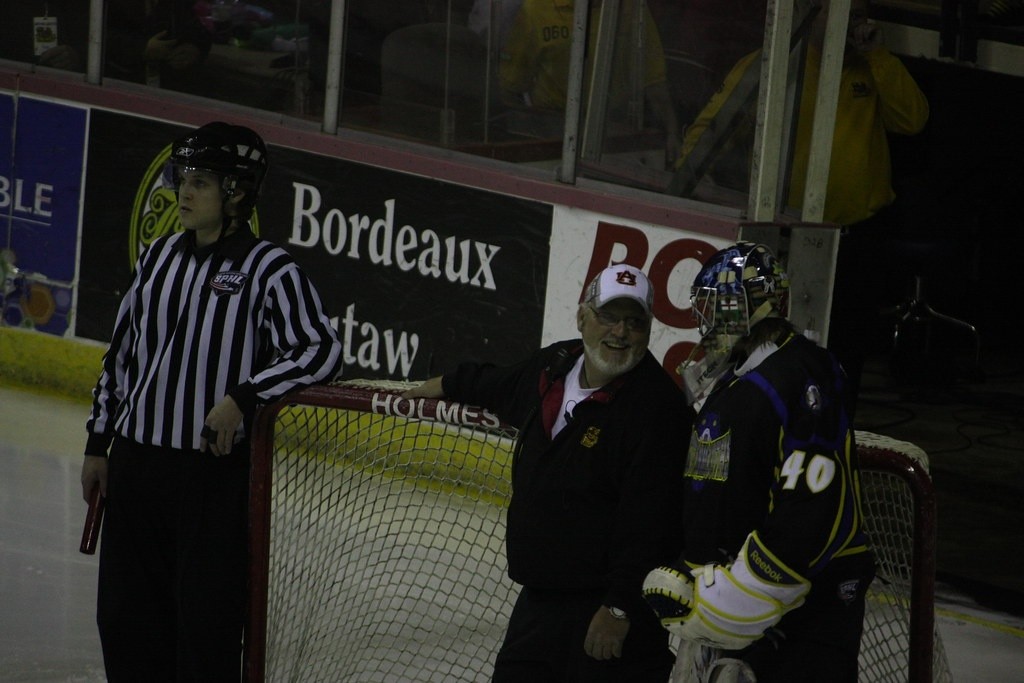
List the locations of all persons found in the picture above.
[673,0,930,420]
[400,263,682,683]
[643,243,878,683]
[82,0,683,163]
[80,120,342,683]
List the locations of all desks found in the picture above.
[584,153,803,229]
[280,102,664,160]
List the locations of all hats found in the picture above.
[584,264,654,320]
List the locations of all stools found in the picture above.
[876,229,989,392]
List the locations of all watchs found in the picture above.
[608,606,626,619]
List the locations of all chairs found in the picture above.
[654,2,765,125]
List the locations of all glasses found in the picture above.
[589,307,649,333]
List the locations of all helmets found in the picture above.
[163,120,268,199]
[690,245,789,378]
